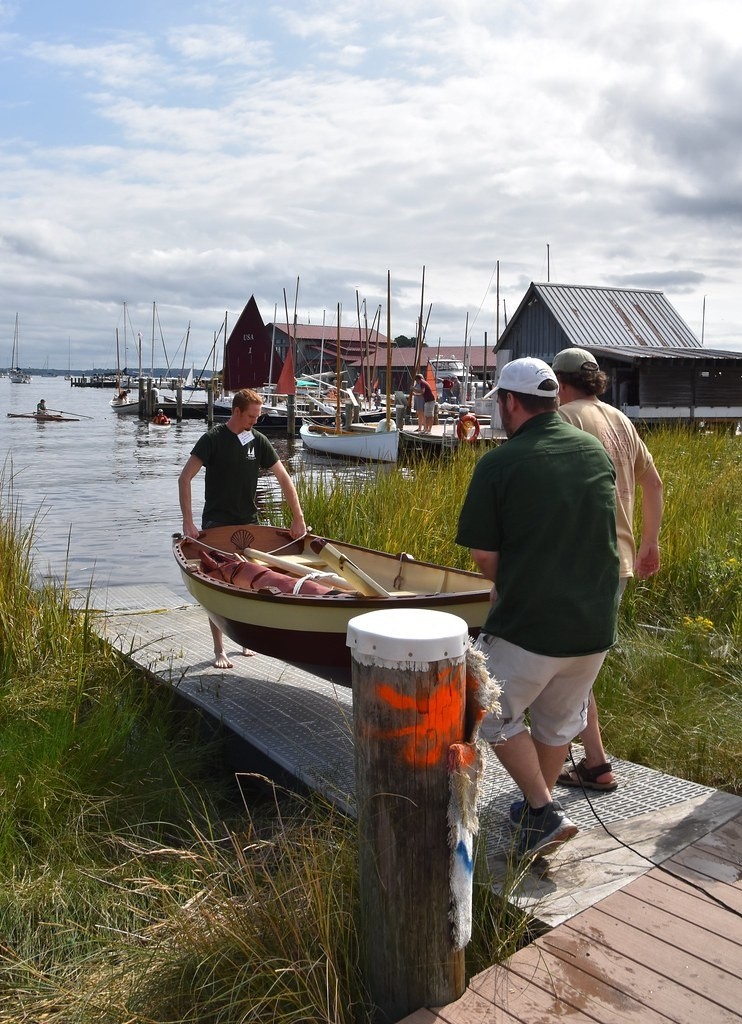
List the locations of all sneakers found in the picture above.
[510,800,529,828]
[515,801,580,862]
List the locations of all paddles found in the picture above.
[44,407,95,421]
[5,409,44,418]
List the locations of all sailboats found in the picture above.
[7,312,32,385]
[64,335,74,381]
[118,263,473,464]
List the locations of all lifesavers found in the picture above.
[456,414,480,444]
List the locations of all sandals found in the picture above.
[557,757,619,791]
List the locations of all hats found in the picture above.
[552,348,599,374]
[416,373,423,378]
[484,357,559,398]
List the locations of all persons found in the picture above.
[118,390,131,405]
[36,399,46,415]
[552,347,665,790]
[413,381,426,432]
[413,374,436,435]
[455,357,622,858]
[178,388,307,668]
[152,409,170,425]
[437,375,461,404]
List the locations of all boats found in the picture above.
[171,524,494,672]
[7,409,81,423]
[42,372,59,378]
